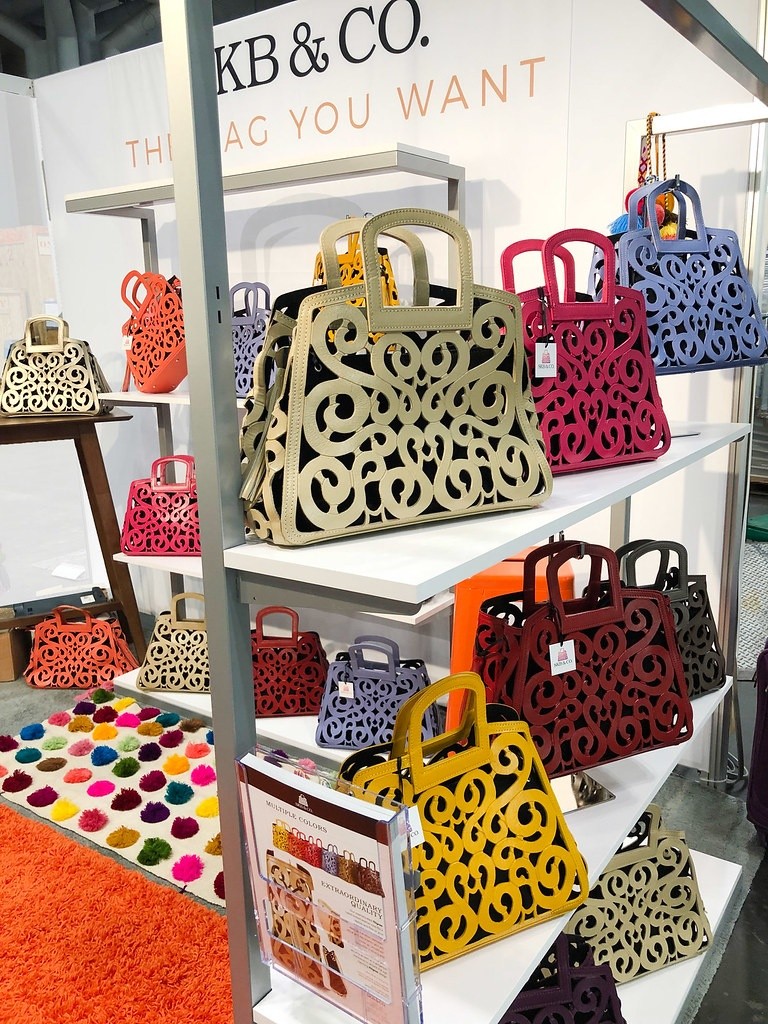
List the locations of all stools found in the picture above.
[442,545,574,756]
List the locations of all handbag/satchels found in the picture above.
[542,803,715,988]
[588,180,767,376]
[121,271,188,394]
[230,282,276,399]
[239,208,554,549]
[120,456,203,556]
[335,672,590,975]
[616,538,726,701]
[313,214,402,352]
[264,816,385,998]
[499,228,672,477]
[136,592,210,695]
[249,606,330,720]
[315,634,444,755]
[22,604,139,691]
[1,314,114,418]
[499,932,626,1024]
[457,539,694,779]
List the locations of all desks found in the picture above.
[0,405,148,667]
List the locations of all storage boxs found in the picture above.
[0,630,32,682]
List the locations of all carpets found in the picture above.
[0,801,234,1024]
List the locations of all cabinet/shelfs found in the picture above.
[68,141,466,773]
[156,0,768,1024]
